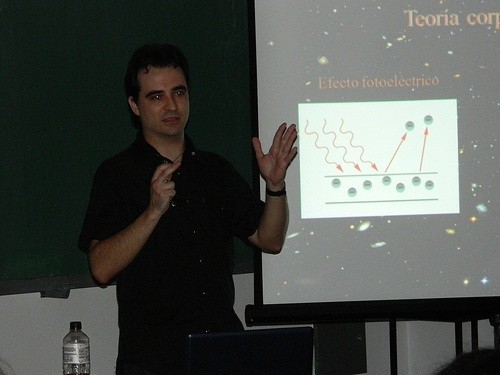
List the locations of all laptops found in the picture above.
[186,326,315,375]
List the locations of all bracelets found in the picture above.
[265,182,287,197]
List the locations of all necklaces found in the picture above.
[171,152,183,161]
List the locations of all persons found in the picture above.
[77,48,299,375]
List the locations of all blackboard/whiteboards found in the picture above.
[0,0,260,304]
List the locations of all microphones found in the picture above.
[165,149,197,207]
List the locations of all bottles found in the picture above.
[63,322,90,375]
